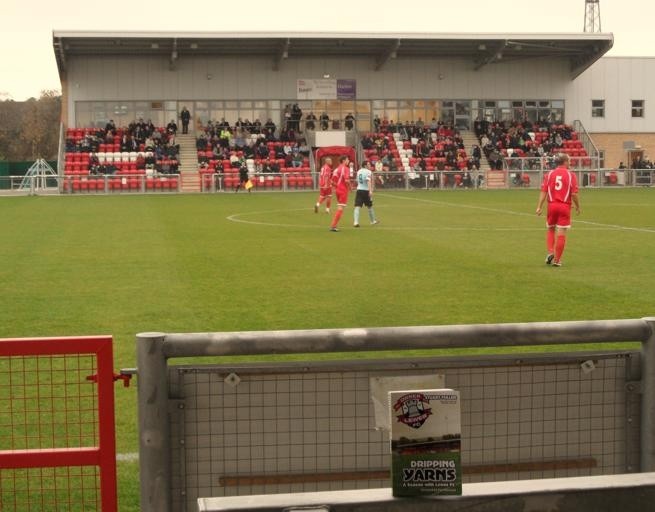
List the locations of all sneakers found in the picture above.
[553,263,561,265]
[546,253,554,264]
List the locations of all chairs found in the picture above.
[360,130,468,183]
[64,126,178,190]
[197,140,309,188]
[525,122,592,169]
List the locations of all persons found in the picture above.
[345,112,356,130]
[314,156,332,213]
[320,112,329,131]
[330,155,352,232]
[66,119,182,177]
[361,115,574,187]
[195,104,317,193]
[536,154,581,267]
[181,106,190,134]
[353,161,376,227]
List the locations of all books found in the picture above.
[388,389,462,497]
